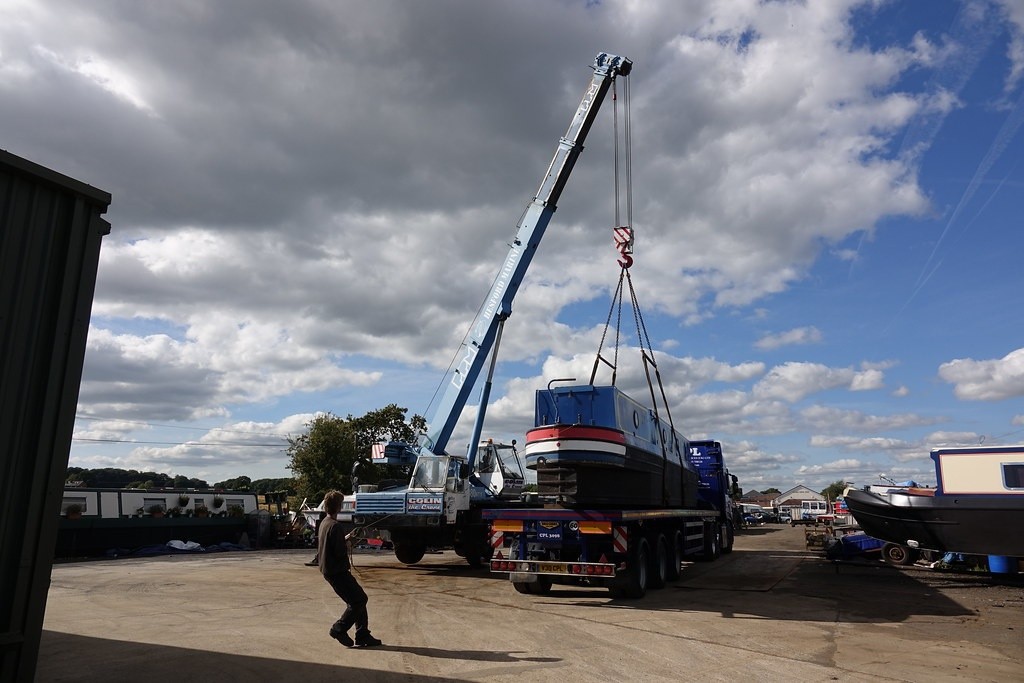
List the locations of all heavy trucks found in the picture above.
[479,438,740,599]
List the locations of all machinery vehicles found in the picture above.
[350,51,689,566]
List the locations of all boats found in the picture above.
[301,494,353,531]
[842,436,1024,556]
[524,378,700,510]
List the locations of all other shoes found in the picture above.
[329,629,354,646]
[355,635,381,646]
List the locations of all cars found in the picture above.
[743,511,792,526]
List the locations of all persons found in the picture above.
[320,490,382,646]
